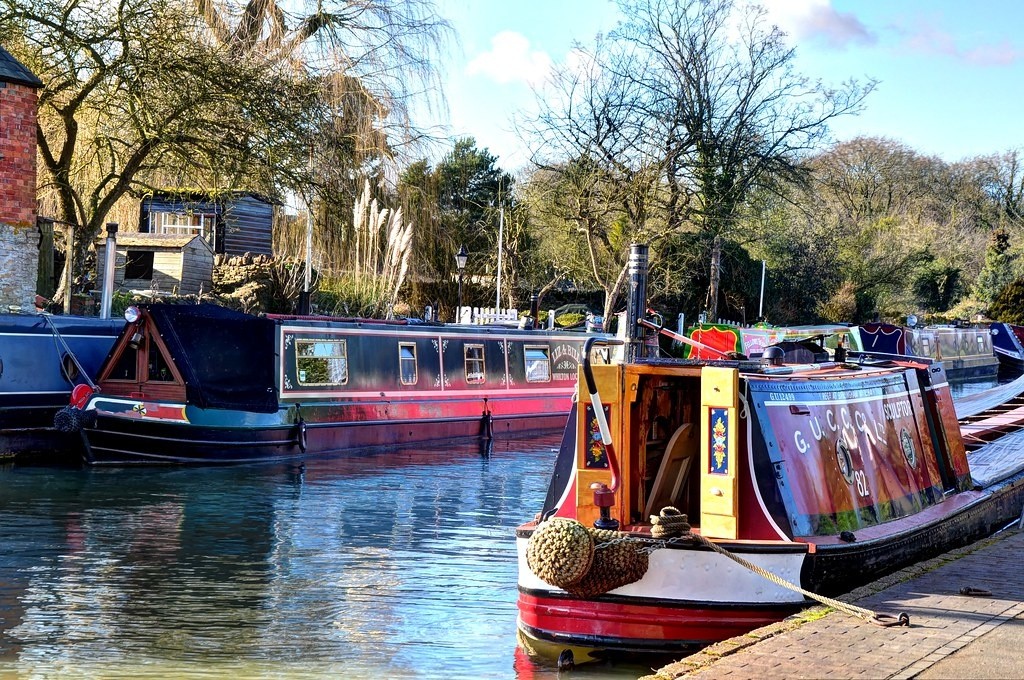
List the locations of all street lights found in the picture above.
[453,244,470,323]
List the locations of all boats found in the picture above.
[683,317,864,359]
[514,243,1023,658]
[53,299,626,464]
[849,315,1001,379]
